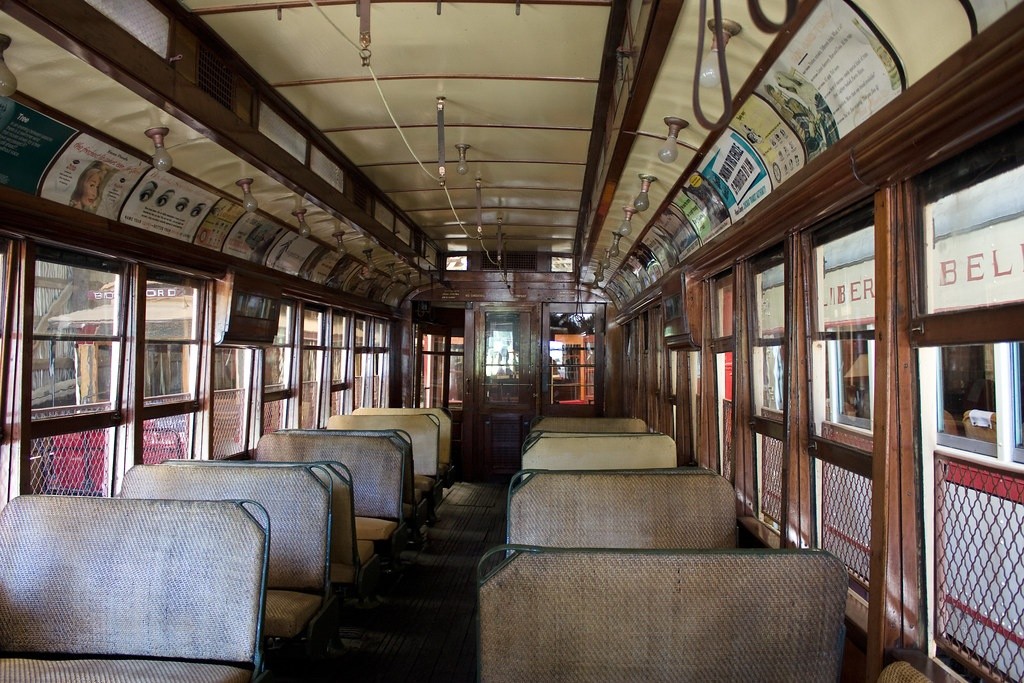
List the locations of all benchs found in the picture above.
[0,407,450,683]
[476,416,964,683]
